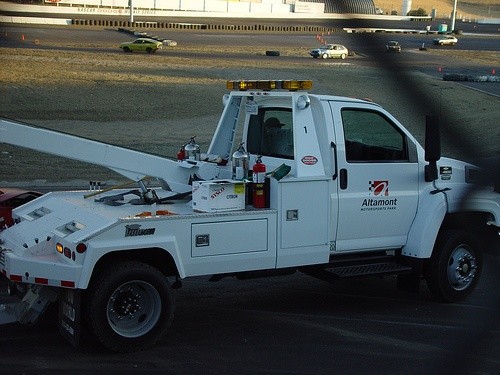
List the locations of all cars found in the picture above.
[385,41,401,53]
[119,38,163,53]
[309,43,348,59]
[433,35,458,46]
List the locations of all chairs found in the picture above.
[265,127,293,155]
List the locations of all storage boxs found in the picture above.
[192,179,246,213]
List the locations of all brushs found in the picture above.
[242,162,291,181]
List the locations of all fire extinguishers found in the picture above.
[252,154,266,208]
[231,142,250,180]
[184,135,201,161]
[177,145,186,160]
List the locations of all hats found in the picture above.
[264,117,285,127]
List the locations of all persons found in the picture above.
[263,117,285,150]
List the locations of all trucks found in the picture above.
[0,79,500,356]
